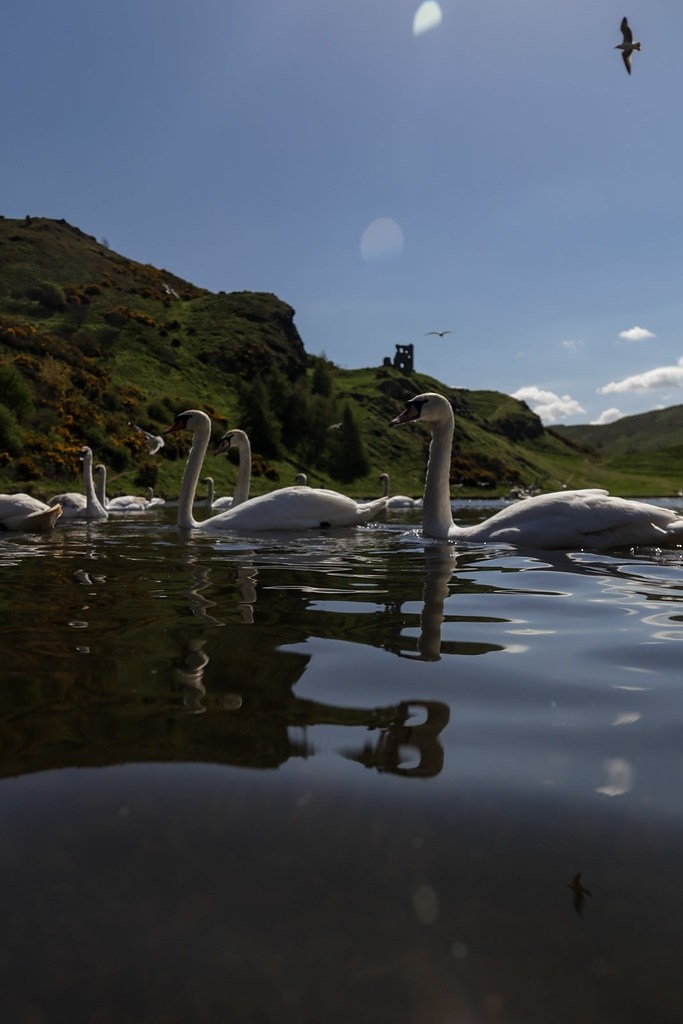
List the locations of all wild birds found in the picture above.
[614,17,641,75]
[424,331,452,337]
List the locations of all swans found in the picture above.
[1,393,683,552]
